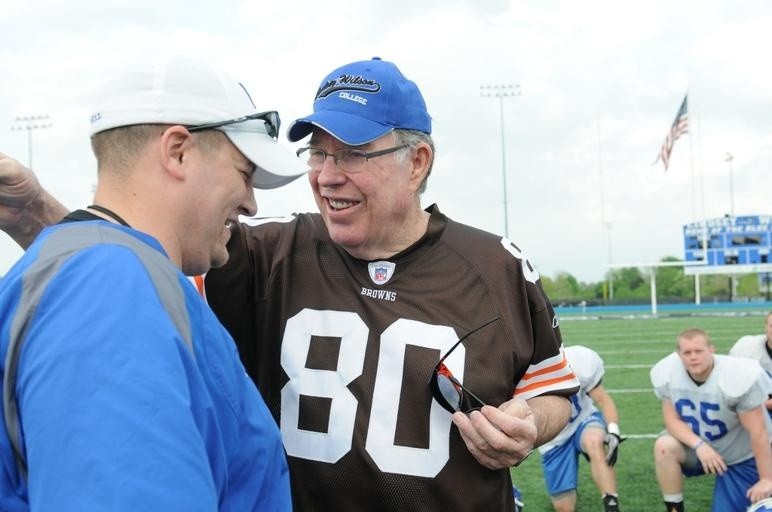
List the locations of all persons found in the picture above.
[726,312,772,412]
[648,326,772,511]
[534,341,626,512]
[1,71,311,511]
[3,53,578,512]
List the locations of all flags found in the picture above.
[658,93,688,169]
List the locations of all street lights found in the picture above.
[477,81,526,241]
[4,110,56,180]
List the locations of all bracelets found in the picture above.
[691,440,706,452]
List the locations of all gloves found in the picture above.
[604,433,627,467]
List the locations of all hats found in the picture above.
[90,75,312,189]
[287,57,431,147]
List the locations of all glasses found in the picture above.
[161,111,280,142]
[428,315,502,414]
[297,137,409,173]
